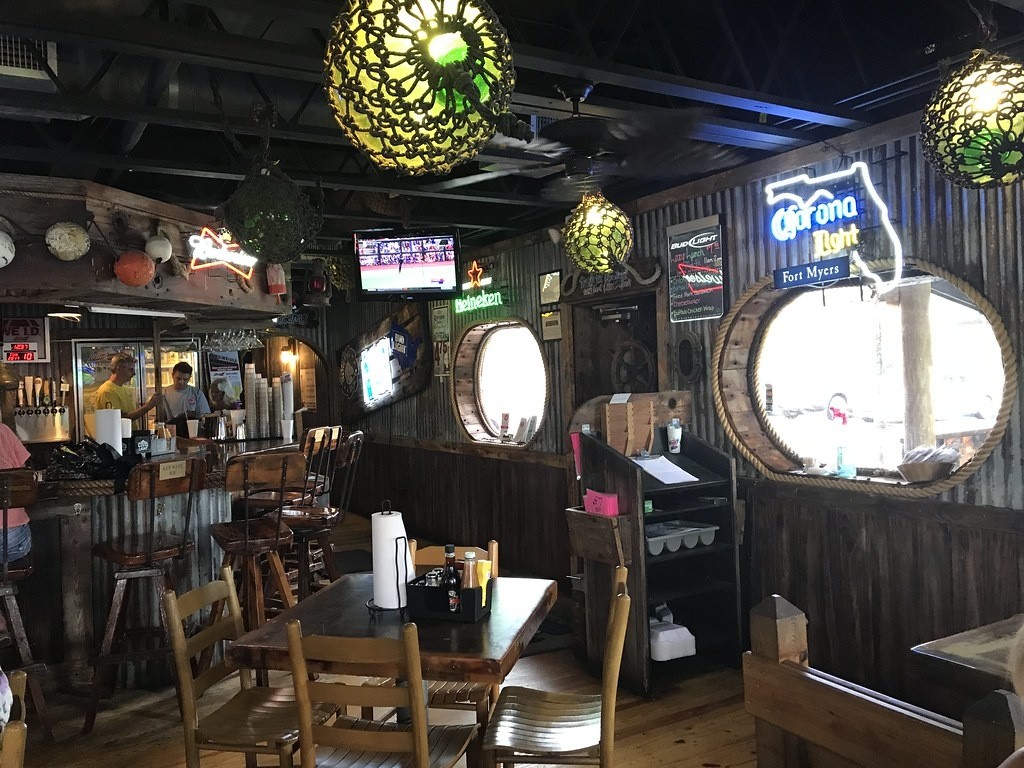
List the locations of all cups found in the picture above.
[281,420,294,438]
[245,364,283,439]
[223,410,246,426]
[667,427,682,453]
[205,415,225,440]
[803,458,816,473]
[187,420,199,438]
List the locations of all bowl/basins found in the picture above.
[897,463,956,482]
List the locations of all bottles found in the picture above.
[148,414,166,439]
[444,545,461,613]
[461,552,480,589]
[146,370,194,385]
[432,568,444,584]
[425,573,440,587]
[145,346,193,366]
[225,423,233,439]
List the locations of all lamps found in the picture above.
[281,337,299,363]
[226,161,313,259]
[321,0,517,177]
[918,44,1024,190]
[564,188,635,274]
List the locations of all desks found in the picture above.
[225,566,558,726]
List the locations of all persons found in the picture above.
[209,379,241,412]
[95,352,165,431]
[0,422,32,650]
[162,362,211,421]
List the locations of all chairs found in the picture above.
[1,425,629,768]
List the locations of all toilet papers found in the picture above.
[369,510,416,610]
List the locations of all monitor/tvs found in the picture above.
[355,229,463,301]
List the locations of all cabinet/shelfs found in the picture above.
[577,430,747,690]
